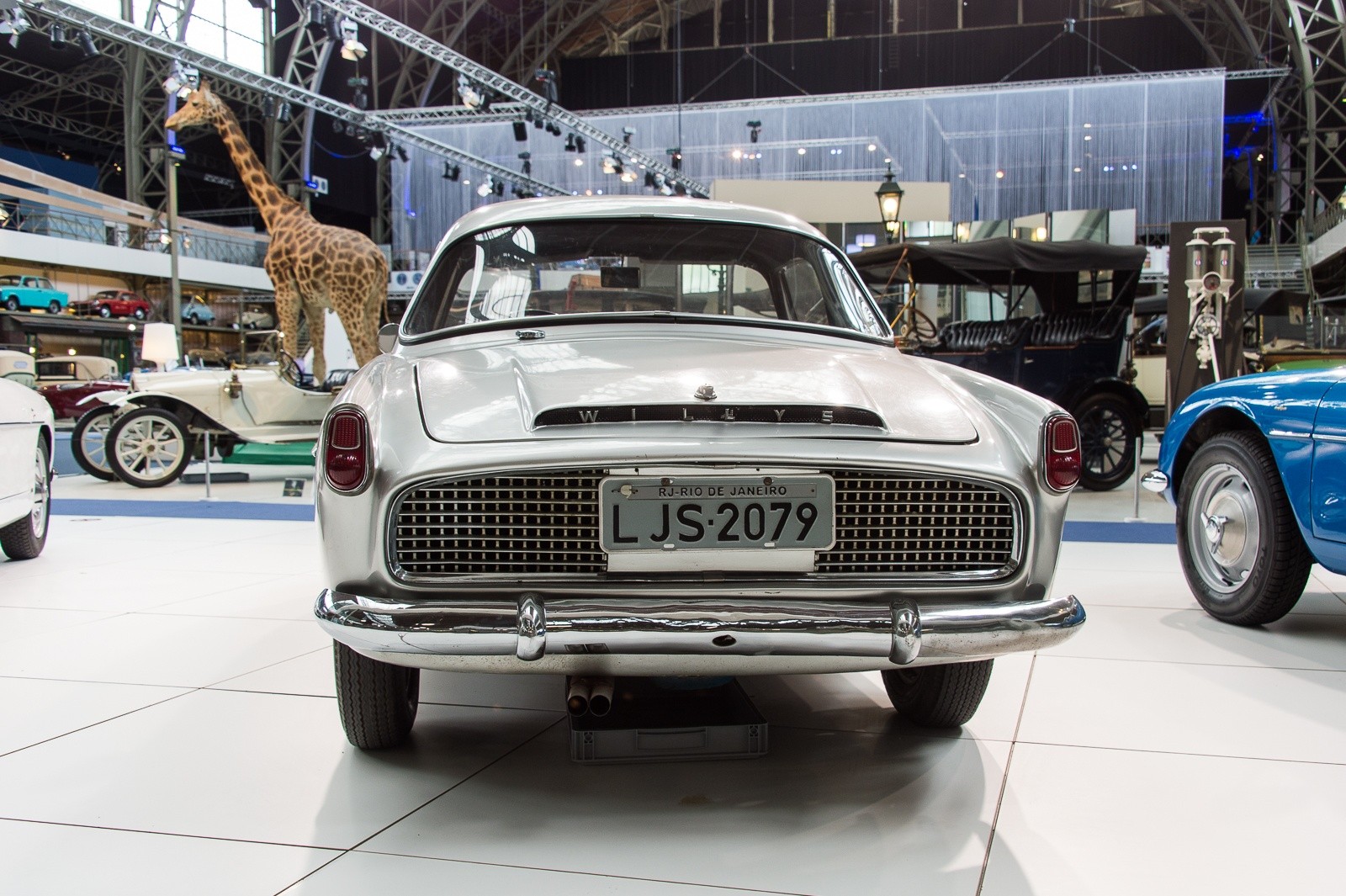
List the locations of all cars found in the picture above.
[31,355,131,424]
[226,304,274,330]
[68,290,150,321]
[0,273,69,314]
[71,327,358,489]
[0,376,57,562]
[1140,365,1346,627]
[158,293,217,326]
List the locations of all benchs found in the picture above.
[941,312,1108,350]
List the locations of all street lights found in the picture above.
[873,161,904,336]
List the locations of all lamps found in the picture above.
[490,177,534,199]
[458,73,501,111]
[76,29,98,56]
[333,119,408,162]
[323,10,341,41]
[304,1,323,29]
[525,106,561,136]
[564,132,576,152]
[603,126,709,199]
[747,121,762,142]
[276,102,292,123]
[261,92,275,118]
[876,162,903,236]
[49,22,67,49]
[440,161,460,181]
[162,59,200,98]
[354,93,367,110]
[575,135,586,153]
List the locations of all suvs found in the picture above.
[1130,287,1313,440]
[310,193,1088,754]
[749,236,1148,487]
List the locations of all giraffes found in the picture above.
[162,77,392,390]
